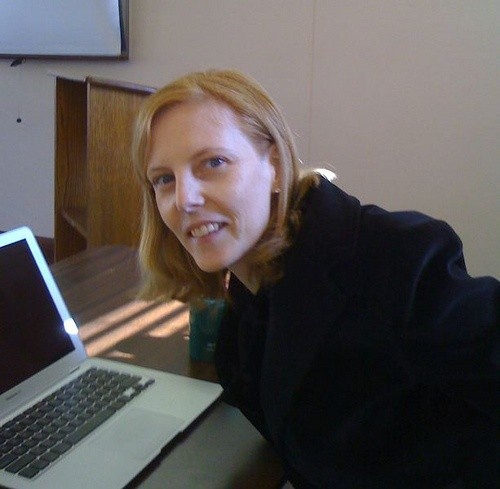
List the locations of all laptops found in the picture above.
[1,226,223,489]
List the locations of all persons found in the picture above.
[134,69,499,488]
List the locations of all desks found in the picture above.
[47,245,231,385]
